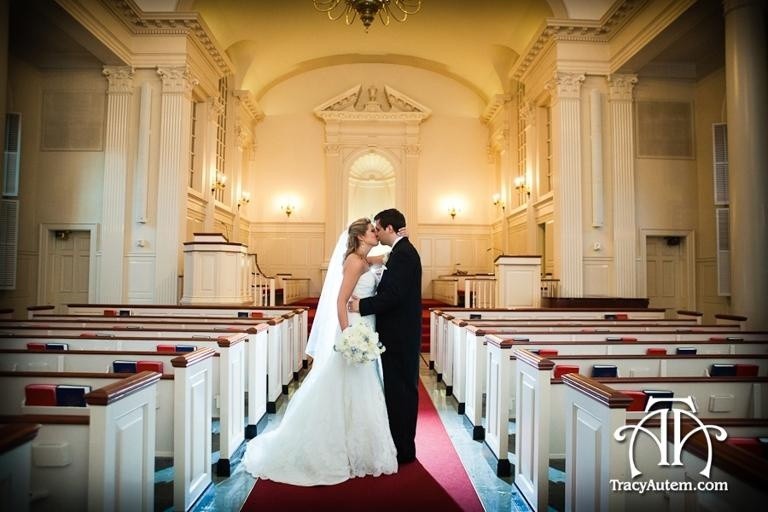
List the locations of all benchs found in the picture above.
[1,302,309,512]
[426,305,768,512]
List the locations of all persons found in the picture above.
[346,208,423,465]
[303,217,411,479]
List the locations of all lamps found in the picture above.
[312,1,425,35]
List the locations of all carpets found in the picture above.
[239,374,486,512]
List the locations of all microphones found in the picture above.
[487,247,504,255]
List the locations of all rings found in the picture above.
[348,306,351,310]
